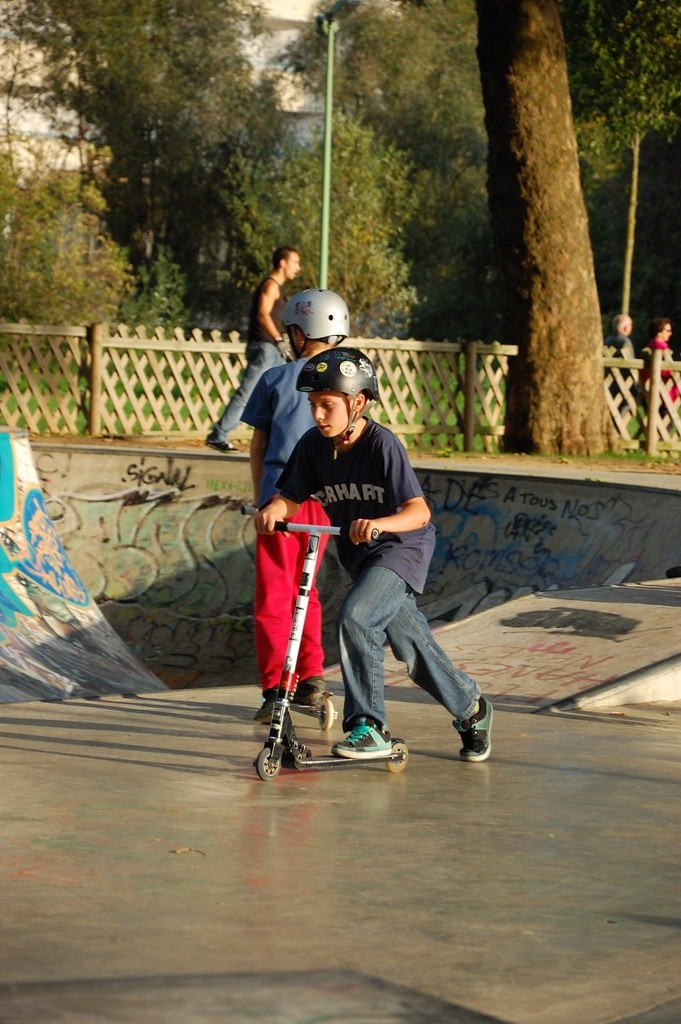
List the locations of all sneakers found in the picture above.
[452,697,494,762]
[332,716,392,759]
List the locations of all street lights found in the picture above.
[317,14,342,292]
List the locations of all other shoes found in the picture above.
[206,436,239,453]
[254,689,282,723]
[289,680,324,705]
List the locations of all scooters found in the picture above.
[252,521,409,782]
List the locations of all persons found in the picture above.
[205,246,301,454]
[603,314,636,411]
[639,319,679,432]
[239,288,350,723]
[253,347,494,761]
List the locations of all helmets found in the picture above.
[282,289,349,339]
[296,347,379,400]
